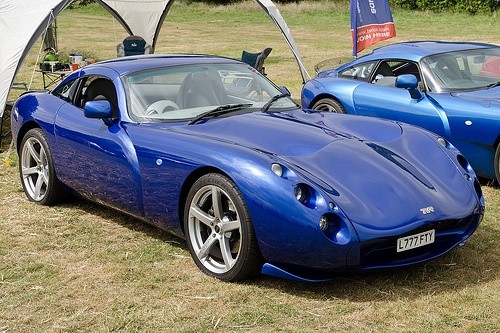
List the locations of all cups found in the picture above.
[72,64,78,72]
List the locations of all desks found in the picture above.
[35,67,73,90]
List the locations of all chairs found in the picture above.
[368,62,397,87]
[10,82,28,91]
[116,35,153,57]
[434,55,463,83]
[176,69,227,110]
[82,78,119,117]
[241,47,272,76]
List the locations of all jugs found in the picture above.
[43,47,60,60]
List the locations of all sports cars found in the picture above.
[9,52,485,283]
[300,41,500,185]
